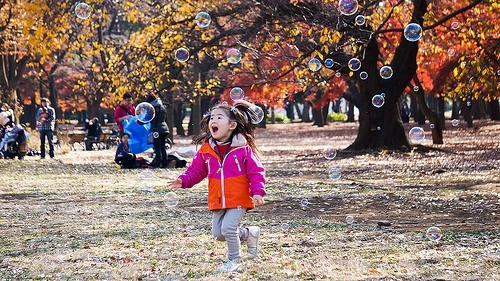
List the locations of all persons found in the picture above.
[114,132,136,169]
[134,90,170,168]
[36,97,55,159]
[167,102,267,272]
[0,103,26,159]
[85,117,102,150]
[113,92,136,138]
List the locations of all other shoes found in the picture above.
[247,226,260,258]
[217,259,245,272]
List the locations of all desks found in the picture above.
[61,129,113,151]
